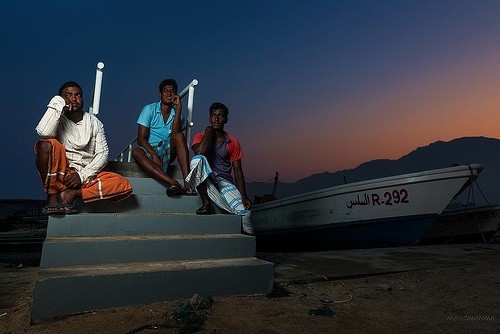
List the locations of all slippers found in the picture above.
[42,203,64,214]
[61,204,79,214]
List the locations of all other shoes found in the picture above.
[182,187,198,196]
[196,204,214,215]
[166,180,182,197]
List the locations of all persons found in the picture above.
[184,102,255,234]
[33,80,133,216]
[131,79,198,197]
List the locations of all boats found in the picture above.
[250,163,483,251]
[420,196,500,244]
